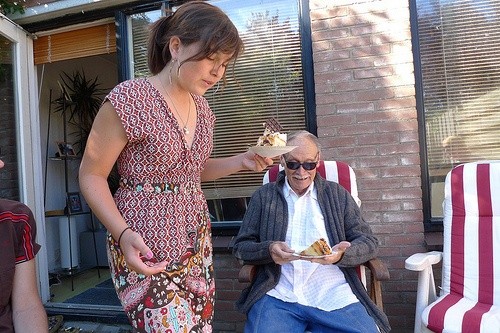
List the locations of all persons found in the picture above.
[0,1,272,333]
[235,130,393,333]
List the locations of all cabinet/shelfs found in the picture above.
[44,90,101,290]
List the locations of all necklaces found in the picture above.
[157,72,190,135]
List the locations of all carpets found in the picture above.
[64,278,122,306]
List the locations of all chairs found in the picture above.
[404,160,500,333]
[239,160,391,312]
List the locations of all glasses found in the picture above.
[283,152,319,170]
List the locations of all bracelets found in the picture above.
[118,226,131,247]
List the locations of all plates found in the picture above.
[249,146,299,159]
[292,251,338,259]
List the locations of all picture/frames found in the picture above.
[56,142,76,156]
[66,192,82,213]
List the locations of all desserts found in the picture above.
[300,238,332,256]
[256,117,288,146]
